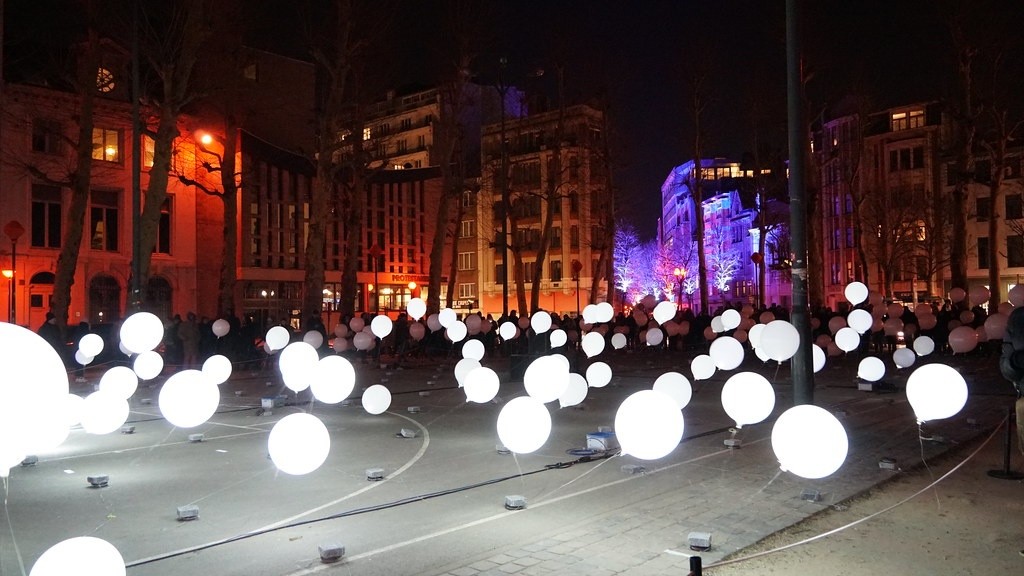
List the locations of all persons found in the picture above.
[37,304,988,375]
[1001,307,1024,461]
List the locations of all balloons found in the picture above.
[885,285,1024,424]
[164,315,393,483]
[203,355,233,384]
[709,336,744,371]
[614,283,886,478]
[455,362,591,459]
[361,385,392,415]
[406,296,629,391]
[99,366,137,400]
[847,309,872,334]
[949,326,977,355]
[531,311,552,334]
[760,320,800,365]
[721,372,774,427]
[913,335,934,357]
[1,313,164,576]
[266,326,290,351]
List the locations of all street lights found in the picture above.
[674,266,686,310]
[370,244,383,314]
[3,220,26,323]
[572,260,583,315]
[1,266,16,322]
[408,281,417,320]
[460,56,544,318]
[750,252,762,309]
